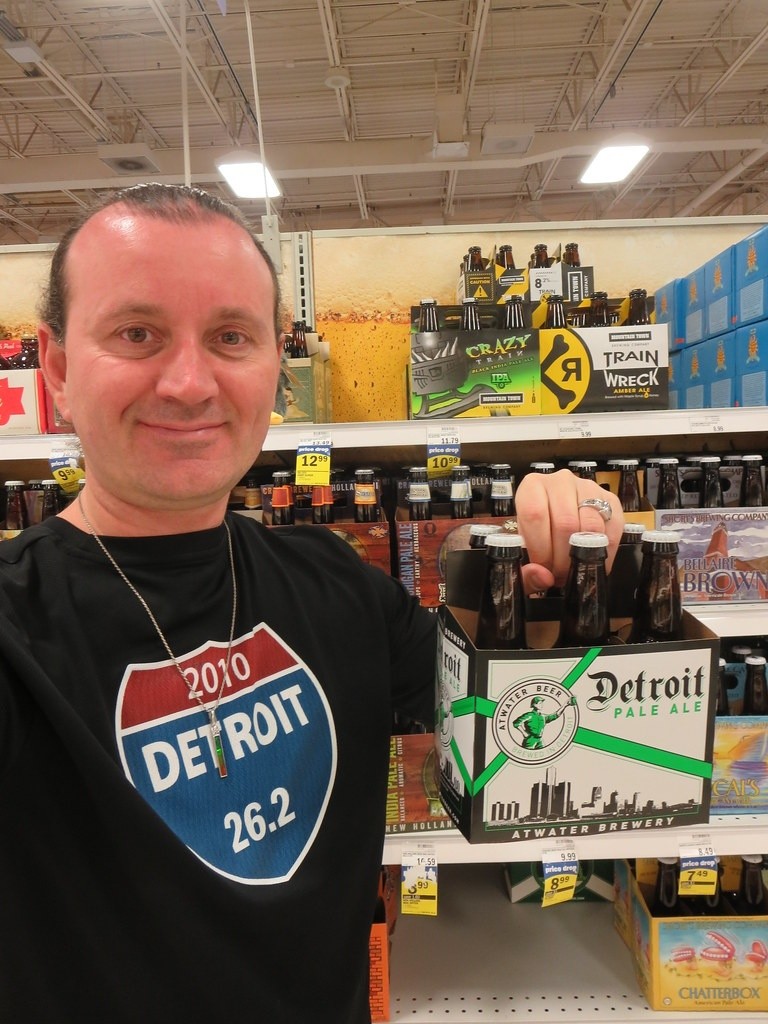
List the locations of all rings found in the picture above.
[578,499,612,521]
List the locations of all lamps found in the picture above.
[577,132,654,184]
[214,149,283,199]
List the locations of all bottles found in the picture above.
[273,471,295,525]
[551,531,626,649]
[739,855,765,916]
[609,523,646,618]
[290,320,308,358]
[703,857,724,917]
[409,467,432,520]
[530,455,768,508]
[450,466,474,519]
[243,477,263,510]
[469,524,503,549]
[491,464,513,516]
[473,533,531,650]
[312,485,333,524]
[419,289,651,332]
[718,646,768,715]
[649,857,683,916]
[463,243,580,272]
[0,334,39,371]
[355,469,377,523]
[0,479,86,530]
[624,530,685,644]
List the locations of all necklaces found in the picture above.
[78,491,237,776]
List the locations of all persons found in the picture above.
[0,182,623,1024]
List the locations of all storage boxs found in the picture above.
[632,881,768,1012]
[708,715,768,815]
[573,469,655,535]
[610,856,742,950]
[385,732,458,835]
[434,550,719,844]
[393,480,519,608]
[263,497,391,578]
[645,466,768,606]
[0,225,768,435]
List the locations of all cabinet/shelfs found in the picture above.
[0,406,768,866]
